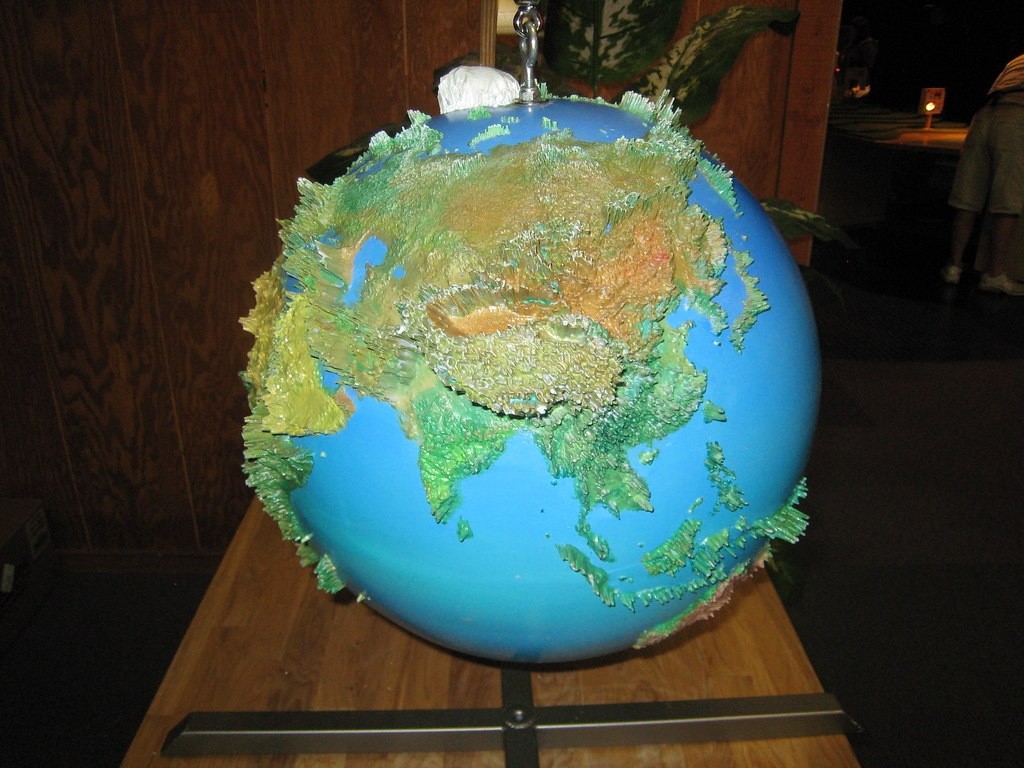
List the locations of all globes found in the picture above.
[159,17,851,760]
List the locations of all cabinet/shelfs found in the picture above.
[1,1,844,575]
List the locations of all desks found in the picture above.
[118,491,863,768]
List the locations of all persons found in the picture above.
[938,54,1024,296]
